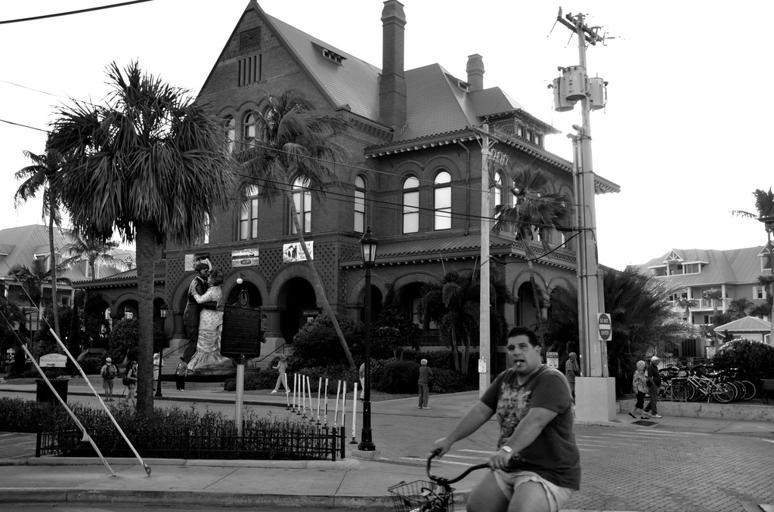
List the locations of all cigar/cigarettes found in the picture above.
[499,360,522,390]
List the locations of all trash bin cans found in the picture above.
[34,379,68,404]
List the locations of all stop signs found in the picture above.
[595,312,612,342]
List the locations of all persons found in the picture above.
[269,354,290,396]
[358,362,367,400]
[174,363,189,390]
[121,360,138,409]
[431,325,581,512]
[185,268,234,370]
[643,357,665,418]
[628,360,650,420]
[152,352,163,393]
[562,350,579,402]
[418,359,434,408]
[99,357,117,402]
[178,264,222,362]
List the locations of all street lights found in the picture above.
[154,305,168,398]
[359,226,377,452]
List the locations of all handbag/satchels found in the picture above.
[123,373,136,385]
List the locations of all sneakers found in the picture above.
[176,388,185,392]
[104,398,116,401]
[270,389,291,394]
[417,404,433,410]
[628,409,662,419]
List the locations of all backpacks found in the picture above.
[105,363,117,379]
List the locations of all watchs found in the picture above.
[500,444,515,457]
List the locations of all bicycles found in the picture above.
[389,448,517,511]
[657,361,757,405]
